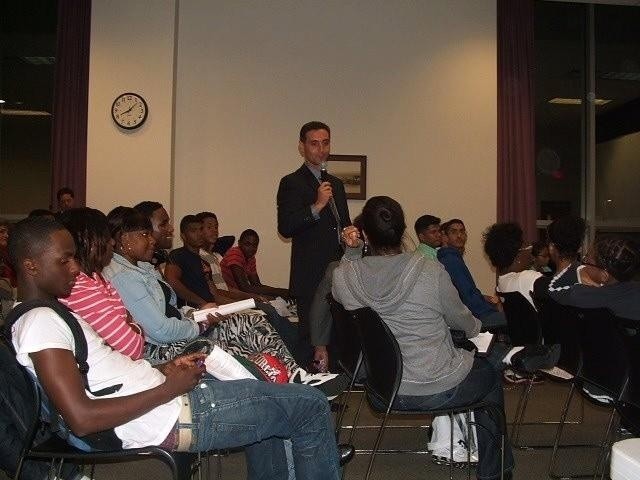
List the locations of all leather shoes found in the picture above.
[511,344,562,371]
[339,445,354,467]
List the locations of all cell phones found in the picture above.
[195,357,205,368]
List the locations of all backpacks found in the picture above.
[0,300,122,450]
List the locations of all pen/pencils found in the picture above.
[310,359,320,364]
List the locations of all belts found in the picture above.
[177,393,192,453]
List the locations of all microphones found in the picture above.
[320,162,328,182]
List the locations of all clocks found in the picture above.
[111,93,148,129]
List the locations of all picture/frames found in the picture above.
[328,155,366,200]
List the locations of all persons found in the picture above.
[165,215,238,310]
[220,229,299,325]
[437,220,508,328]
[580,238,640,288]
[485,222,574,379]
[136,201,321,373]
[197,212,269,303]
[54,188,76,219]
[9,217,341,480]
[102,207,344,401]
[277,122,353,410]
[332,196,562,480]
[526,243,552,273]
[0,218,18,302]
[534,216,587,290]
[58,208,297,480]
[28,209,55,221]
[414,215,443,262]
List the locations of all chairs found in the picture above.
[327,292,466,452]
[344,306,505,480]
[0,331,200,480]
[510,290,600,448]
[549,306,640,480]
[495,291,584,423]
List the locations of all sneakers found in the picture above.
[288,368,350,396]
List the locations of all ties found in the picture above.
[319,176,342,235]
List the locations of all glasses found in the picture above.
[533,254,550,257]
[519,244,534,253]
[582,247,611,274]
